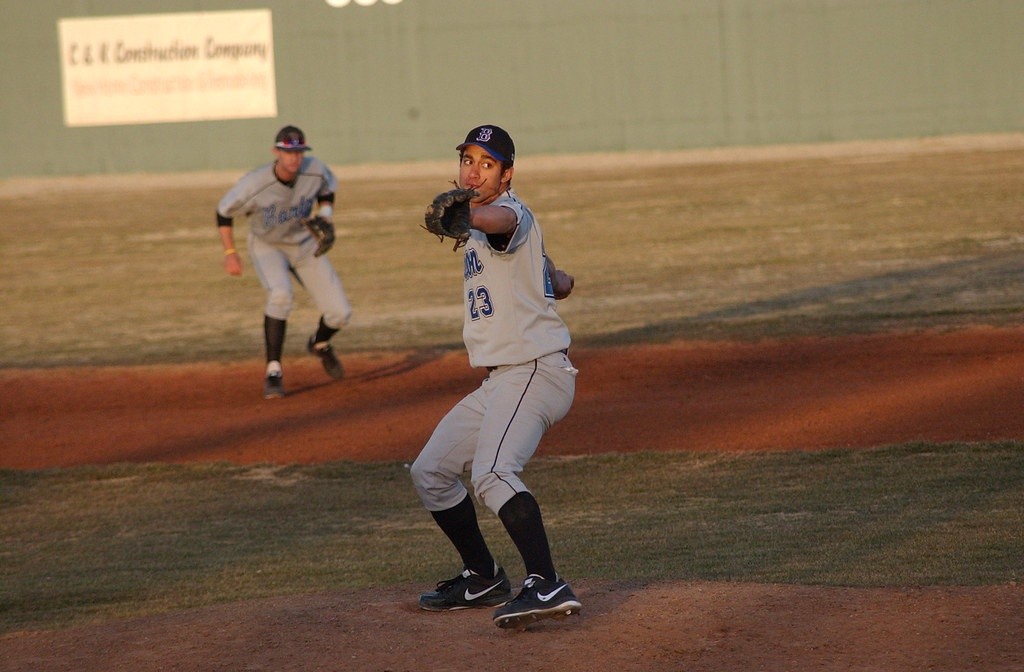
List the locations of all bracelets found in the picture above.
[226,249,236,256]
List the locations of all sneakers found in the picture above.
[419,566,513,612]
[493,572,582,628]
[306,334,345,379]
[264,372,285,400]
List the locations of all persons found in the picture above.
[410,124,583,627]
[217,129,354,400]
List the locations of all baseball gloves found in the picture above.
[418,177,488,252]
[300,214,335,257]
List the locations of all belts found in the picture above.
[486,349,567,372]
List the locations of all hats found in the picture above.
[275,126,312,151]
[456,125,515,167]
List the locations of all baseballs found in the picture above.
[556,270,572,293]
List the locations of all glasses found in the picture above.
[284,137,304,145]
